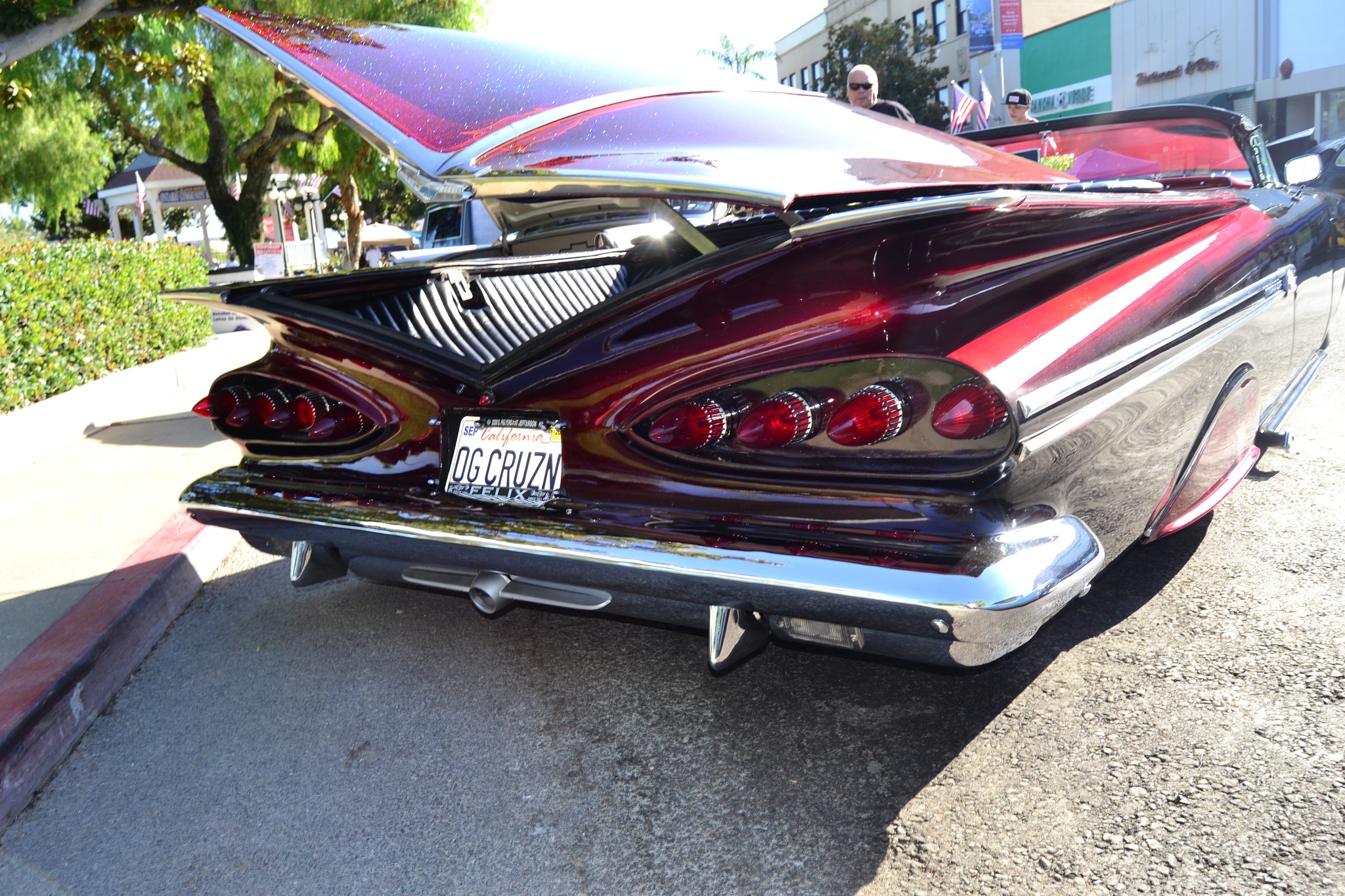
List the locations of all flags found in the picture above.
[282,198,294,218]
[333,184,362,206]
[109,207,122,232]
[231,169,241,201]
[134,171,146,223]
[950,83,977,134]
[975,74,992,131]
[82,198,102,219]
[296,174,327,194]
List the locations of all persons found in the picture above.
[847,63,916,123]
[226,253,240,267]
[1000,88,1038,124]
[359,245,396,268]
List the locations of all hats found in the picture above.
[1000,88,1032,108]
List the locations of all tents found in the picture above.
[337,222,413,271]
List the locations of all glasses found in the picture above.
[848,82,877,91]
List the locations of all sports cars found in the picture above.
[178,4,1344,677]
